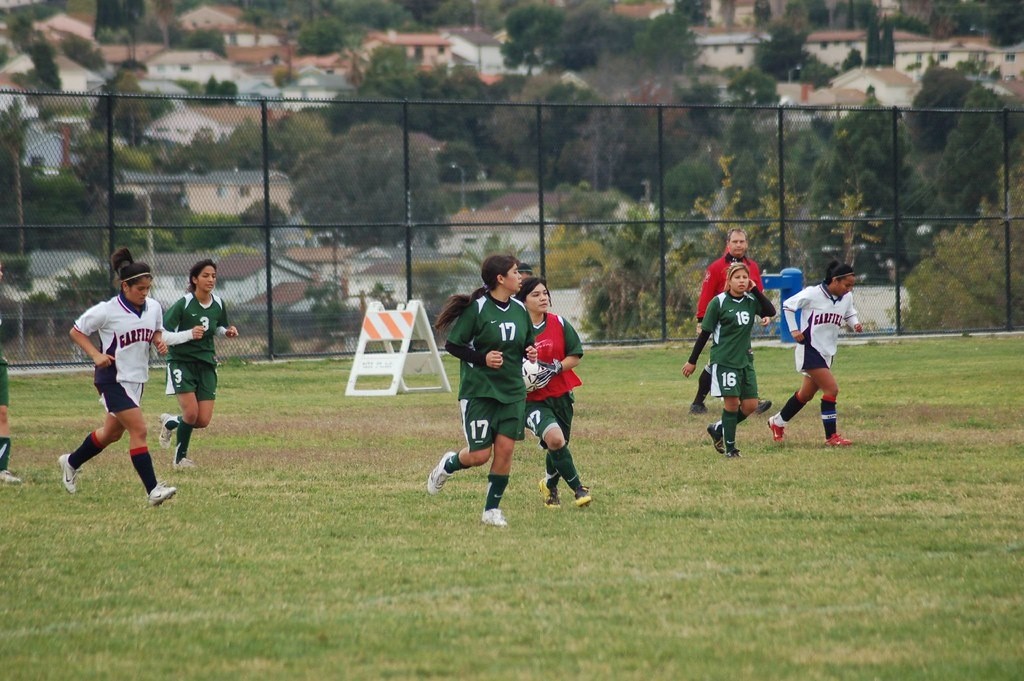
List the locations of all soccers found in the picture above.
[521,358,548,392]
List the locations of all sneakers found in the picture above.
[147,481,176,506]
[539,476,561,509]
[825,432,853,446]
[173,458,198,472]
[707,424,725,454]
[724,447,741,458]
[768,416,785,442]
[482,507,507,527]
[160,413,173,449]
[574,485,592,507]
[58,454,83,493]
[0,470,21,482]
[427,451,457,495]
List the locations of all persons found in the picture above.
[427,254,537,527]
[158,258,239,472]
[768,260,864,447]
[514,276,592,509]
[0,261,22,484]
[682,262,776,458]
[690,227,772,417]
[518,263,533,280]
[56,248,178,507]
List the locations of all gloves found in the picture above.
[535,360,562,390]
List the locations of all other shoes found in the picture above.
[690,402,708,412]
[758,399,772,414]
